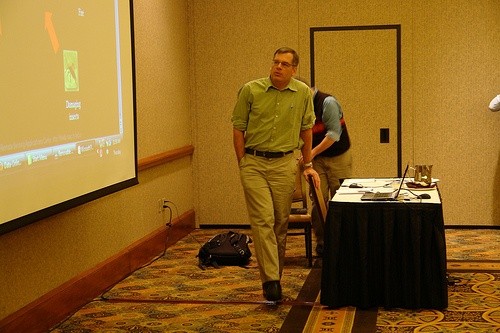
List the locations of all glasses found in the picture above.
[272,60,297,68]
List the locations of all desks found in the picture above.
[320,178,448,311]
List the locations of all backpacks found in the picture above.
[198,231,252,269]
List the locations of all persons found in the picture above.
[294,76,352,268]
[230,47,320,302]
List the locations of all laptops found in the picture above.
[361,164,409,201]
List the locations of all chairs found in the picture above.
[287,159,314,268]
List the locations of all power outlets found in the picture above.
[162,197,167,212]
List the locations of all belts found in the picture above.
[246,148,294,159]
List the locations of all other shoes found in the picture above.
[263,281,281,301]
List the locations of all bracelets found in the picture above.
[305,162,312,168]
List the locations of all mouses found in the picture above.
[417,193,431,199]
[349,183,363,188]
